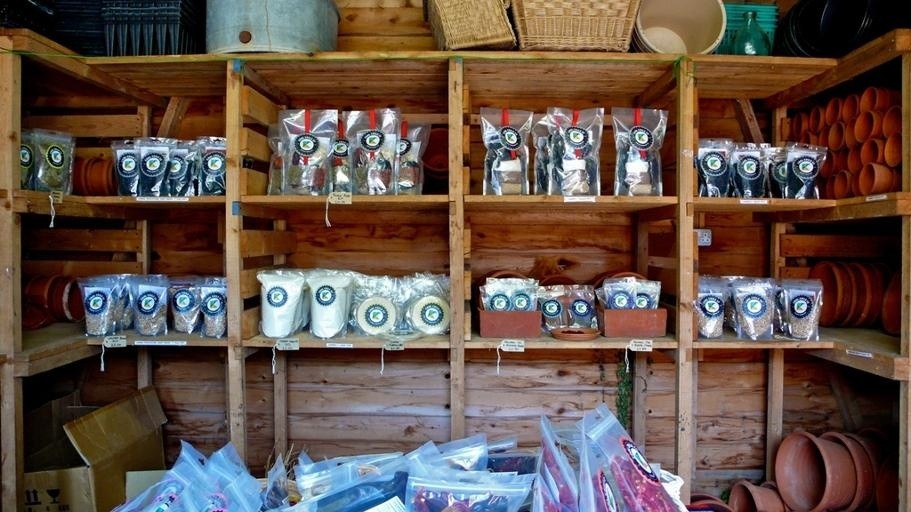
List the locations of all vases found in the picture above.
[24,278,86,329]
[699,88,902,511]
[71,154,122,194]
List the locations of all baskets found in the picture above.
[428,1,517,51]
[509,0,640,51]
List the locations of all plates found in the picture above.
[551,326,603,340]
[488,270,648,291]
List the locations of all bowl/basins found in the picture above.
[806,259,902,335]
[206,0,342,54]
[630,0,727,56]
[776,424,901,511]
[780,1,911,57]
[690,480,789,512]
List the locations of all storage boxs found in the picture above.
[20,386,171,512]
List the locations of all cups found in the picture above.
[793,86,903,199]
[22,278,85,329]
[423,127,449,189]
[73,157,118,196]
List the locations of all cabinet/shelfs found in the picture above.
[0,25,169,511]
[86,49,840,512]
[764,27,909,512]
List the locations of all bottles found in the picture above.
[733,11,772,56]
